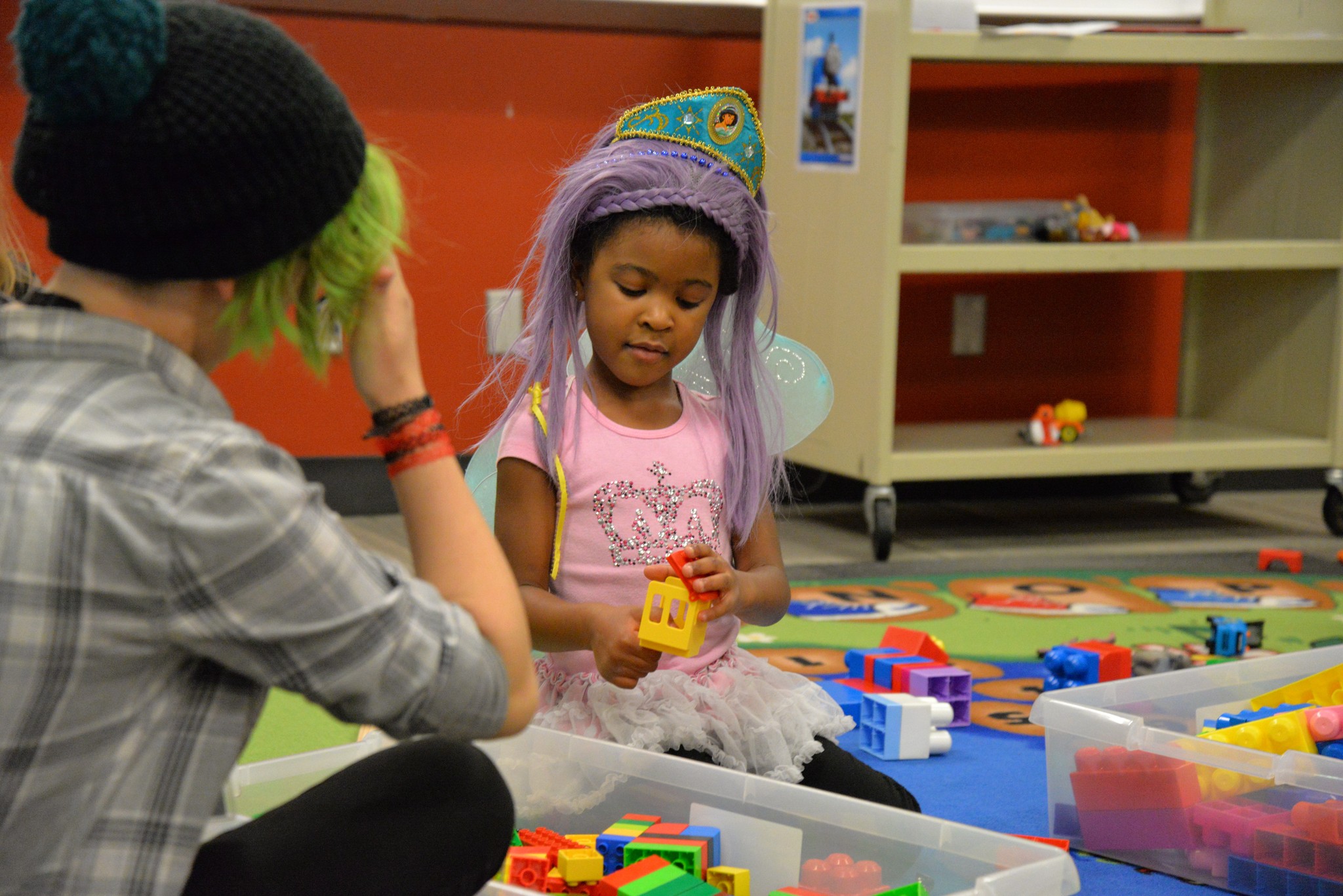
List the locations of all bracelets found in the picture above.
[363,394,457,476]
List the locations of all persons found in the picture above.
[457,86,925,883]
[0,0,540,896]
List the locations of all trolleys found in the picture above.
[752,0,1343,560]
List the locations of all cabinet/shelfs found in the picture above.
[759,0,1343,563]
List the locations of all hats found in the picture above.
[10,1,367,283]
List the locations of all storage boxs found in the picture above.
[1029,627,1343,896]
[200,726,1082,896]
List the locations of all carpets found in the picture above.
[227,572,1343,896]
